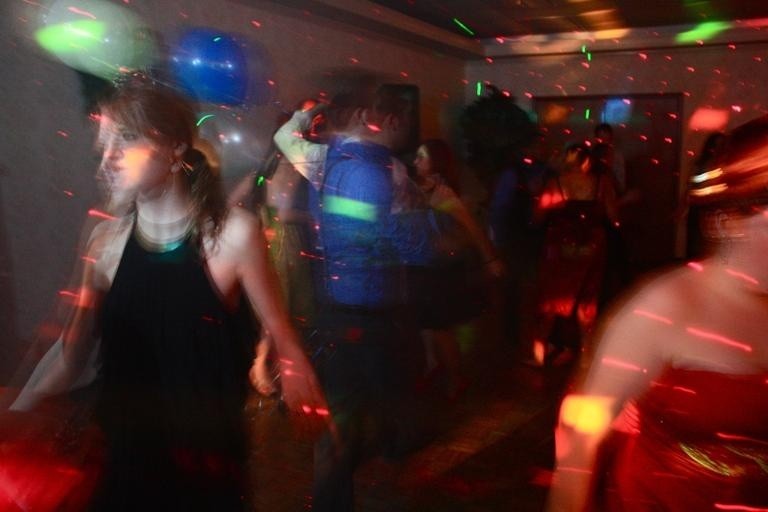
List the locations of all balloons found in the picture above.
[168,26,248,108]
[29,1,156,82]
